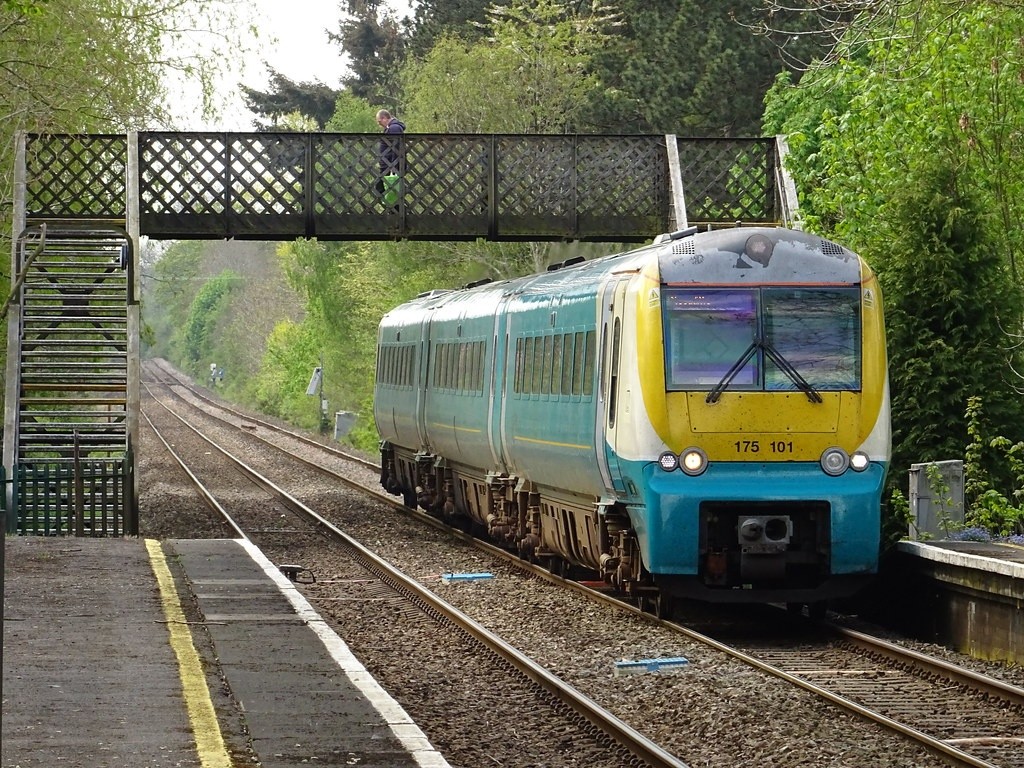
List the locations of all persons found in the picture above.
[218,367,224,381]
[372,108,406,212]
[211,367,217,385]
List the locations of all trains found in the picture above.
[373,221,892,617]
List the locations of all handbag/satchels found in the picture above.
[382,170,399,205]
